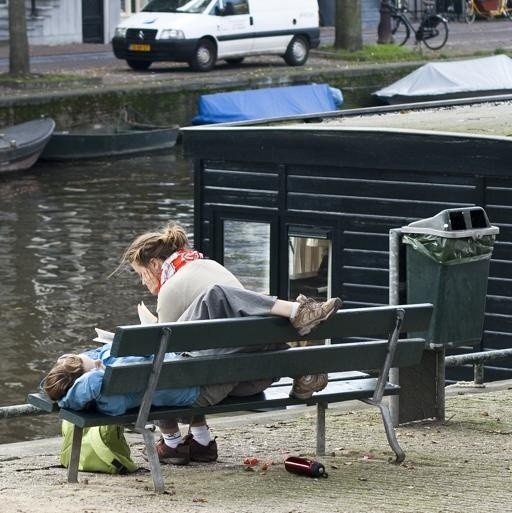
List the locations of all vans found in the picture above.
[112,0,321,71]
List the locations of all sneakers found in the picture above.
[291,294,343,336]
[141,434,218,465]
[289,373,328,400]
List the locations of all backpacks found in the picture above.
[60,419,137,475]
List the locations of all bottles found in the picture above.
[284,457,329,480]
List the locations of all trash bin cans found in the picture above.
[402,206,500,350]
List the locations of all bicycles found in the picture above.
[464,0,512,24]
[391,5,449,50]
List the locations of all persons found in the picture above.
[45,282,342,416]
[125,225,274,465]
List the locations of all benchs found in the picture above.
[28,301,434,493]
